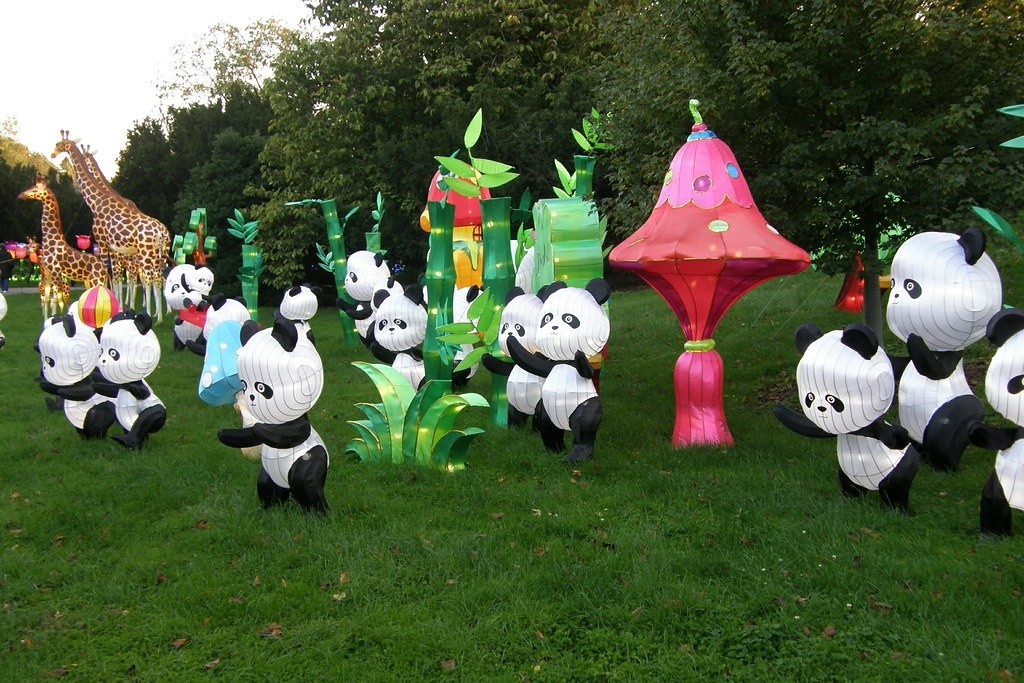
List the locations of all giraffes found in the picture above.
[18,130,175,326]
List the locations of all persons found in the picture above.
[0,246,14,293]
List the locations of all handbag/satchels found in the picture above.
[7,252,15,270]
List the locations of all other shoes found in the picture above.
[2,290,7,293]
